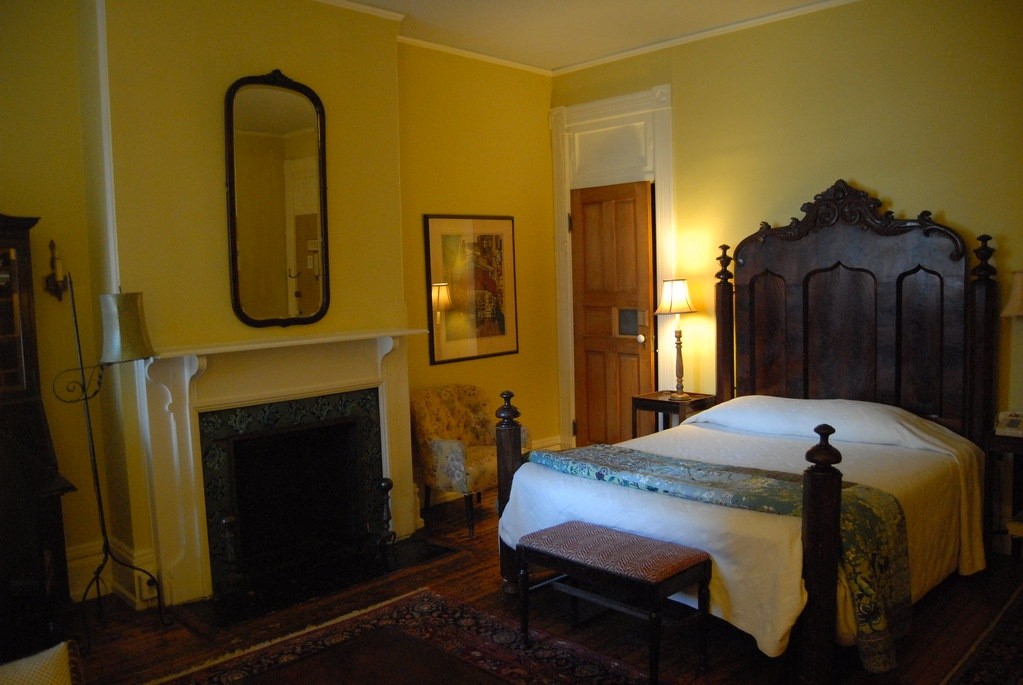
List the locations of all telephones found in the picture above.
[995,410,1023,438]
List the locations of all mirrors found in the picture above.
[223,68,331,328]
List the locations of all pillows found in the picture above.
[0,638,85,685]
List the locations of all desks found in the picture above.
[989,437,1023,558]
[631,390,716,440]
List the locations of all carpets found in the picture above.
[145,585,675,685]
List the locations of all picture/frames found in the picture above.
[423,214,519,366]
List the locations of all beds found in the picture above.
[499,178,1004,685]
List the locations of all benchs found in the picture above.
[515,520,712,685]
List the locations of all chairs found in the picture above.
[406,381,533,542]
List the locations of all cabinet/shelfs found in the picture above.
[0,213,79,622]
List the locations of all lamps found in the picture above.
[44,234,169,626]
[431,283,456,359]
[653,278,699,401]
